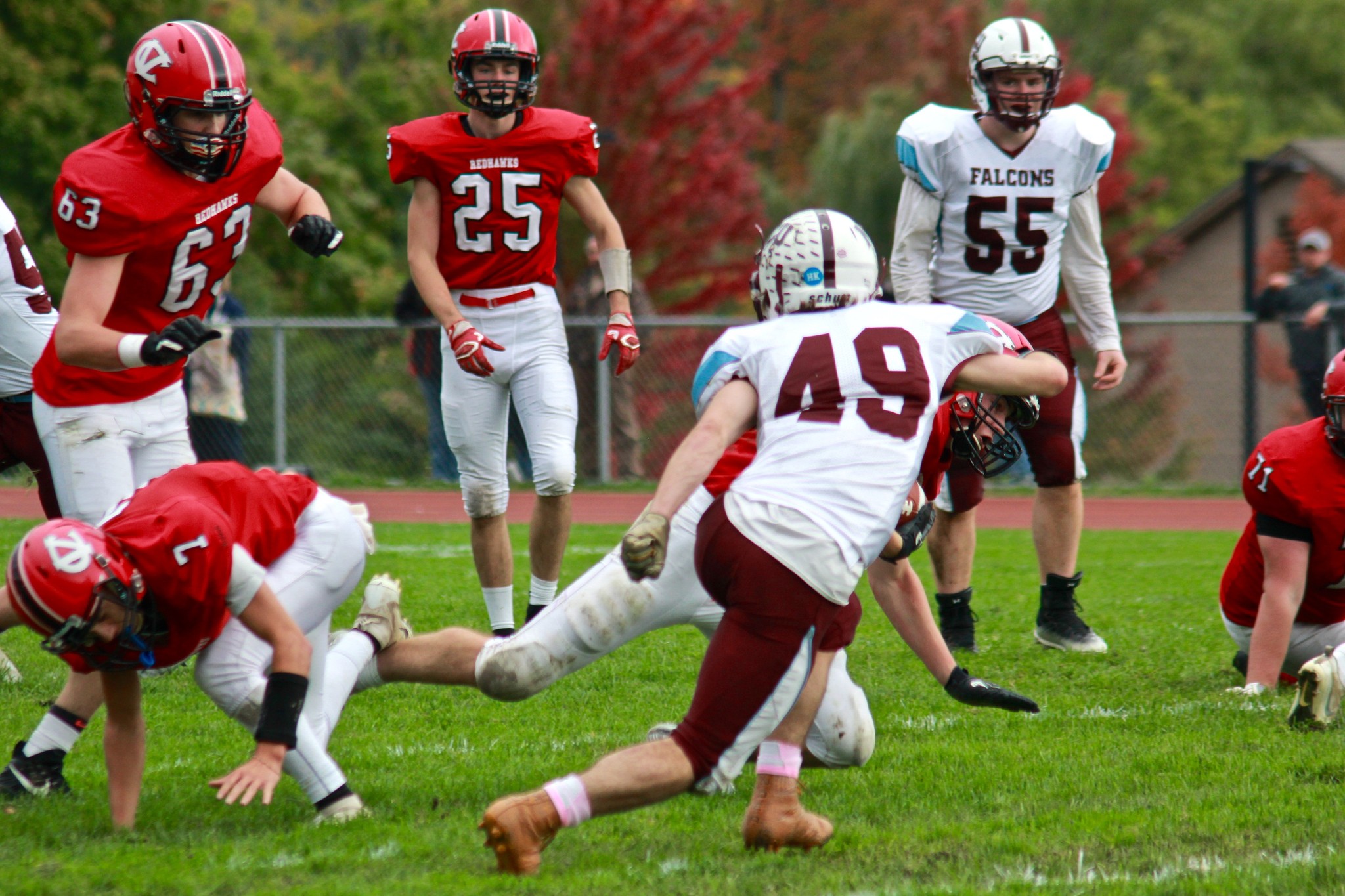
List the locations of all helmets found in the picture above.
[969,17,1065,133]
[751,208,883,321]
[7,518,169,673]
[1321,348,1345,459]
[951,315,1040,476]
[448,8,540,120]
[124,20,253,182]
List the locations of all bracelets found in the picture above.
[252,755,282,777]
[598,248,632,300]
[116,333,150,369]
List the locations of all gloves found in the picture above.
[879,503,936,565]
[621,514,670,583]
[140,315,222,366]
[288,215,344,258]
[598,313,639,376]
[945,667,1041,714]
[446,320,505,378]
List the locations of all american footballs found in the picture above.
[897,478,929,527]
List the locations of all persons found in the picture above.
[179,270,249,468]
[387,9,640,638]
[325,312,1041,794]
[0,195,59,521]
[1218,349,1345,734]
[888,16,1128,654]
[0,20,345,800]
[477,208,1069,877]
[567,233,658,484]
[1253,226,1345,418]
[5,458,414,830]
[390,279,533,485]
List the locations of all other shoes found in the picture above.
[0,651,25,682]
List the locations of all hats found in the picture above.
[1296,230,1332,252]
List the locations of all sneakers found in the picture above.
[934,587,978,653]
[478,789,562,879]
[311,793,372,824]
[0,741,75,802]
[353,573,414,651]
[1287,644,1343,732]
[742,773,833,855]
[1034,572,1108,654]
